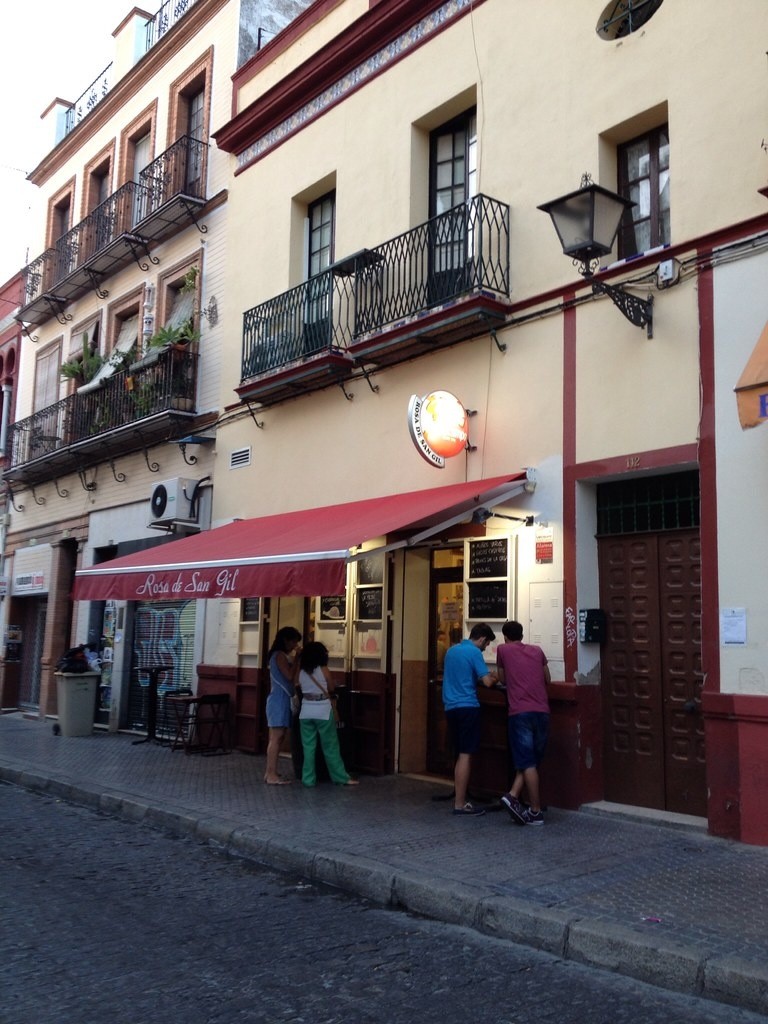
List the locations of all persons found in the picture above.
[498,620,551,825]
[294,641,361,787]
[263,626,302,785]
[442,622,499,817]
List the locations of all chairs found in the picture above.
[160,690,233,756]
[32,424,60,456]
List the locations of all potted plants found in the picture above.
[57,317,202,440]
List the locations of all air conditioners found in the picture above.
[148,477,200,525]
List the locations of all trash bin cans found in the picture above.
[51,670,101,737]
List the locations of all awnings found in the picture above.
[733,320,768,432]
[73,471,528,600]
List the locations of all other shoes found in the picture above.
[345,779,360,785]
[264,774,287,782]
[266,777,291,785]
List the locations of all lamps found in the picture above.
[537,171,654,339]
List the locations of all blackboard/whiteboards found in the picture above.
[355,587,383,621]
[356,549,386,587]
[467,536,509,579]
[319,562,348,621]
[465,580,509,620]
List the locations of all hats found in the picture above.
[471,623,496,641]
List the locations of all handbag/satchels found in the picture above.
[290,694,300,715]
[331,699,340,721]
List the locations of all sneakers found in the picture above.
[511,807,545,826]
[500,793,528,825]
[453,803,485,816]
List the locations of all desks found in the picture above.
[166,696,226,756]
